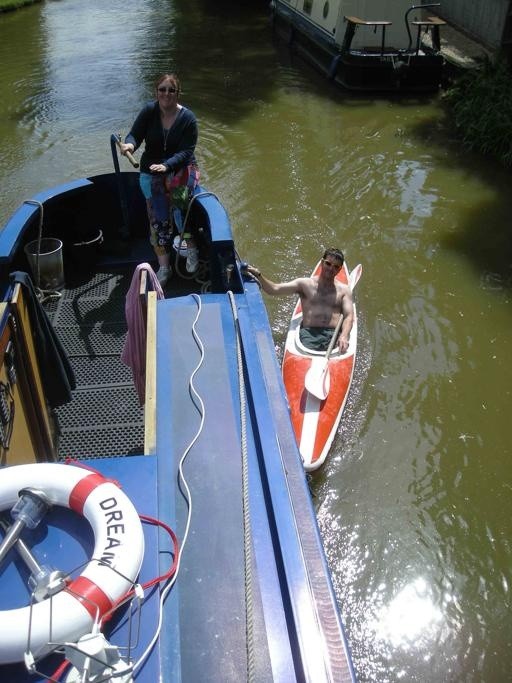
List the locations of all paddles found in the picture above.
[304,262,362,400]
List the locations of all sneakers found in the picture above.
[186,246,200,273]
[156,265,173,287]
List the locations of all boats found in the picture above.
[0,170,360,683]
[282,249,360,470]
[266,0,441,103]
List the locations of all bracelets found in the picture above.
[256,269,262,277]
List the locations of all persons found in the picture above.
[245,245,356,358]
[120,72,201,288]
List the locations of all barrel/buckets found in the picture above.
[65,224,105,271]
[24,237,63,287]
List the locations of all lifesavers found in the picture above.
[1,462,145,664]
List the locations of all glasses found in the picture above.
[157,87,177,93]
[323,260,342,270]
